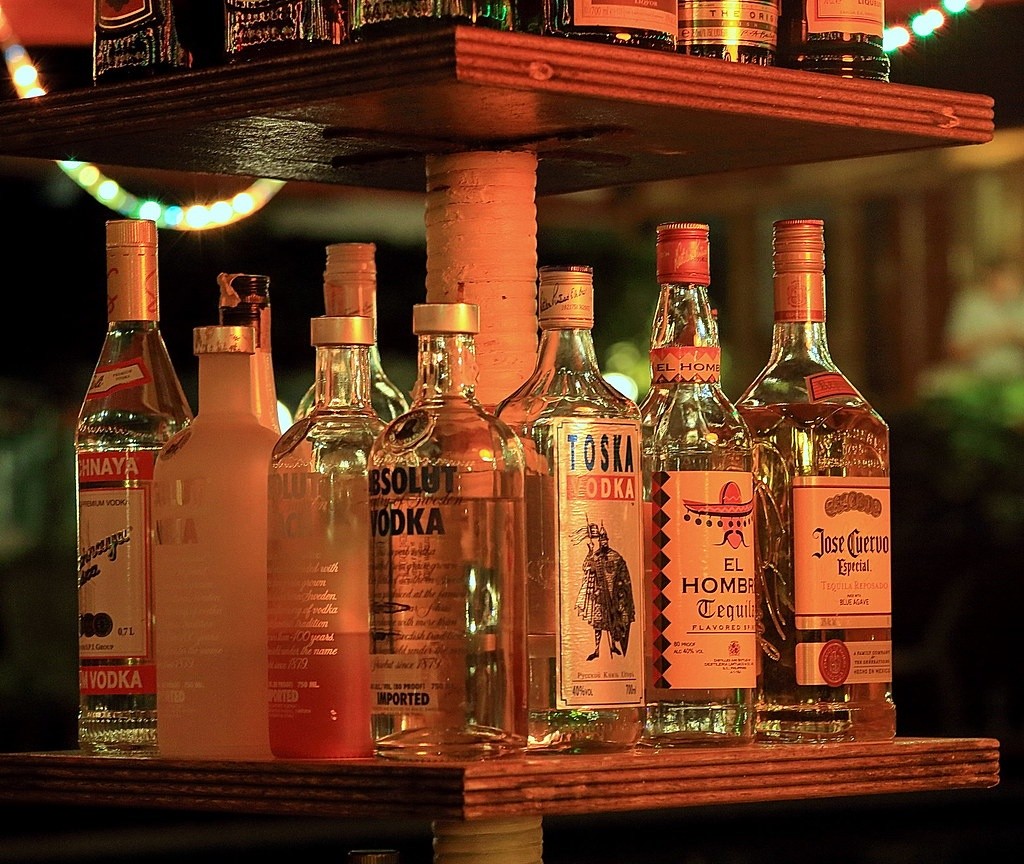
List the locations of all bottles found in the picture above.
[493,264,647,754]
[92,1,891,91]
[735,218,897,745]
[151,327,281,762]
[268,317,391,760]
[294,244,410,424]
[75,219,196,760]
[217,273,280,435]
[638,223,766,744]
[366,301,527,763]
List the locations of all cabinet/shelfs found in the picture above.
[0,27,999,864]
